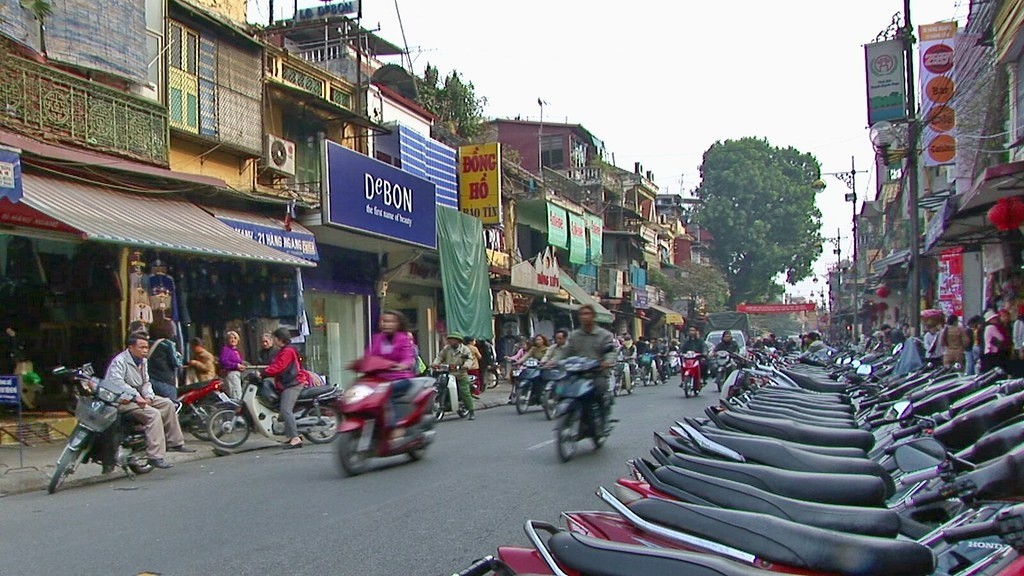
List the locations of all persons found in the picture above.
[99,310,496,472]
[503,303,1024,441]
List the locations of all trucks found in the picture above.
[702,312,750,365]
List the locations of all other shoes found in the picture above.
[166,444,197,453]
[147,459,174,468]
[468,413,475,419]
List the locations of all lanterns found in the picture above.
[988,195,1024,232]
[876,287,891,298]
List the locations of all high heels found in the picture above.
[283,441,303,450]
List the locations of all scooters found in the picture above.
[335,354,442,477]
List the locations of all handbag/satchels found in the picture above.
[414,356,426,375]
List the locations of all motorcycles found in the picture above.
[45,362,168,495]
[204,369,347,449]
[438,326,1024,576]
[428,362,470,423]
[154,377,235,442]
[674,349,709,399]
[534,355,626,464]
[514,358,548,414]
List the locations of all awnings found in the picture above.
[603,202,641,220]
[514,198,583,216]
[602,231,652,245]
[19,172,317,269]
[266,78,393,139]
[558,266,615,323]
[925,160,1024,253]
[651,306,684,325]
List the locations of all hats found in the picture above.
[982,310,999,323]
[446,331,463,341]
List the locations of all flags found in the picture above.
[919,21,959,168]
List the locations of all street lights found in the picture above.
[869,1,926,373]
[802,154,869,346]
[811,227,841,351]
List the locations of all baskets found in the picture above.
[75,396,118,433]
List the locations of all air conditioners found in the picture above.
[258,133,295,177]
